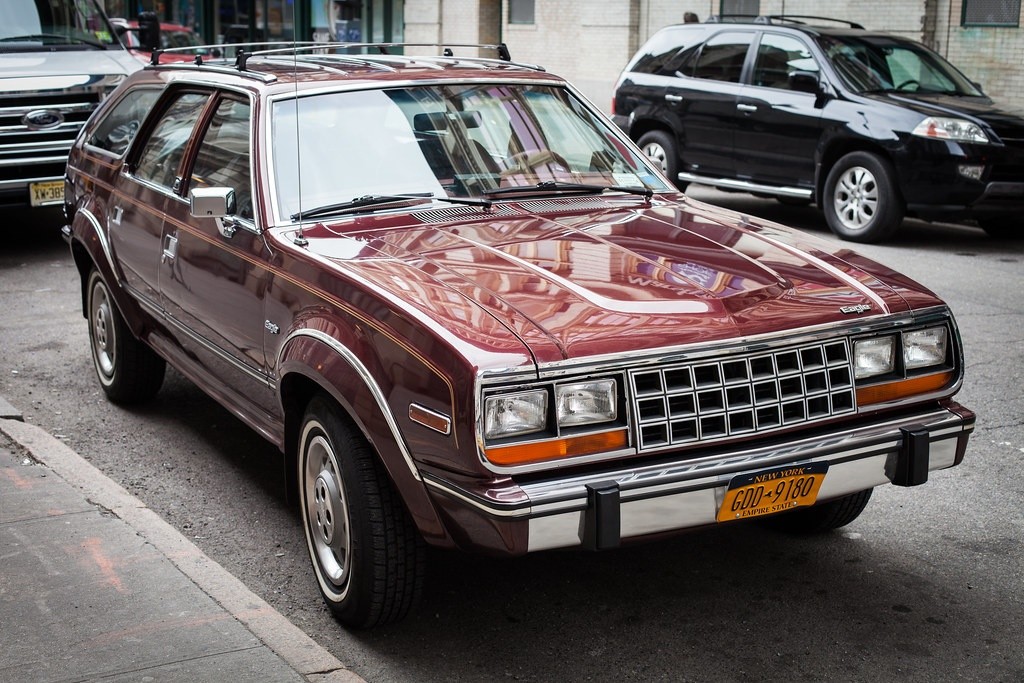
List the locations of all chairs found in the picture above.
[181,110,509,211]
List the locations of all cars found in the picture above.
[57,39,978,636]
[0,0,161,211]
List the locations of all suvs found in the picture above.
[106,16,223,70]
[607,11,1024,248]
